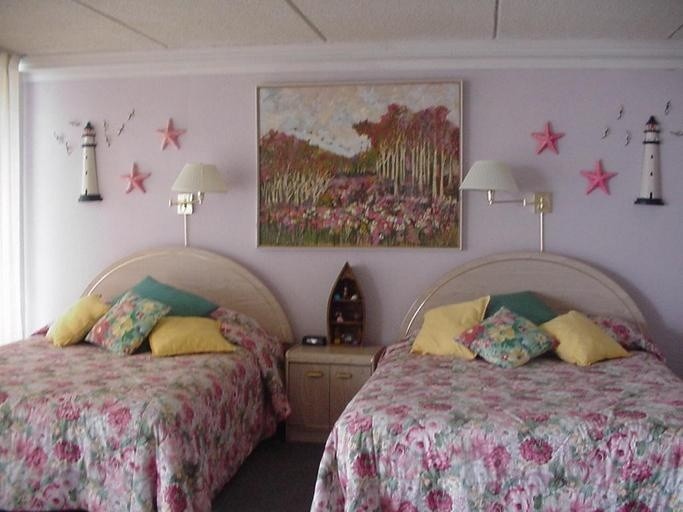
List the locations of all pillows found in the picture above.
[452,306,561,370]
[149,317,236,356]
[85,291,172,355]
[539,311,631,366]
[45,293,111,346]
[484,291,558,327]
[410,295,491,360]
[111,276,220,317]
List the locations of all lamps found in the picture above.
[168,162,227,206]
[458,161,545,206]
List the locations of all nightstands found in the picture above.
[286,343,386,442]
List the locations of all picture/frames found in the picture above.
[255,80,463,250]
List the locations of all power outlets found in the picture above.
[176,194,194,214]
[534,192,552,213]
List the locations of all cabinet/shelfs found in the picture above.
[327,261,366,346]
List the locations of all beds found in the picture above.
[311,253,683,512]
[0,246,294,512]
